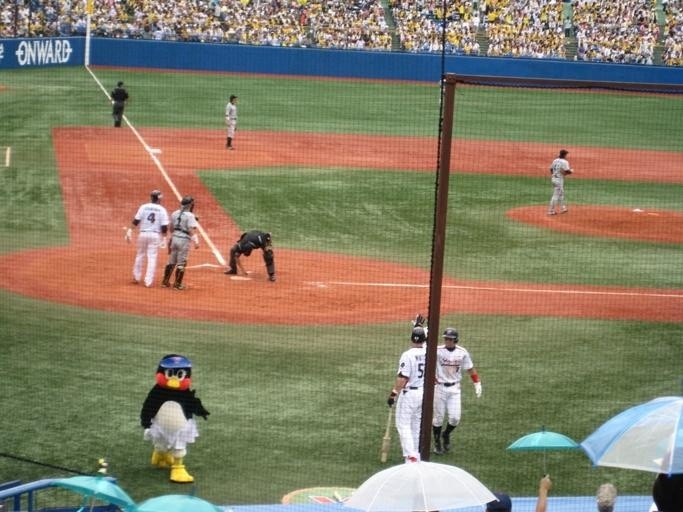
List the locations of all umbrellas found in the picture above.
[135,485,224,512]
[579,395,683,477]
[505,424,581,476]
[49,474,136,512]
[341,451,500,512]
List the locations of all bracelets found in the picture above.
[471,373,479,383]
[163,236,167,241]
[127,229,132,237]
[391,389,398,397]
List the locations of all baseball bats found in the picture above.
[381,406,392,464]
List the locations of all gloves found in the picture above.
[412,314,426,327]
[191,236,199,249]
[387,391,397,408]
[159,238,167,248]
[474,382,482,398]
[125,230,133,243]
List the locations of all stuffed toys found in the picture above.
[140,355,210,483]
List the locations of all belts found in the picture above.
[230,118,236,120]
[444,383,455,386]
[403,387,417,389]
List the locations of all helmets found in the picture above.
[442,329,459,343]
[151,192,162,203]
[412,328,426,342]
[182,197,194,212]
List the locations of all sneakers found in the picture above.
[270,274,276,281]
[443,431,450,450]
[433,443,442,454]
[548,211,556,215]
[224,269,237,274]
[560,209,567,214]
[226,146,234,149]
[404,457,417,463]
[132,277,184,289]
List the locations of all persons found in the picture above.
[387,313,429,462]
[0,0,393,52]
[547,149,575,215]
[159,194,200,290]
[109,81,129,128]
[224,229,276,282]
[432,328,482,456]
[412,446,682,512]
[124,190,171,288]
[224,95,239,148]
[390,0,683,67]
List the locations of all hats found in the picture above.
[487,494,511,511]
[598,484,616,506]
[560,150,568,154]
[118,82,123,87]
[230,95,237,100]
